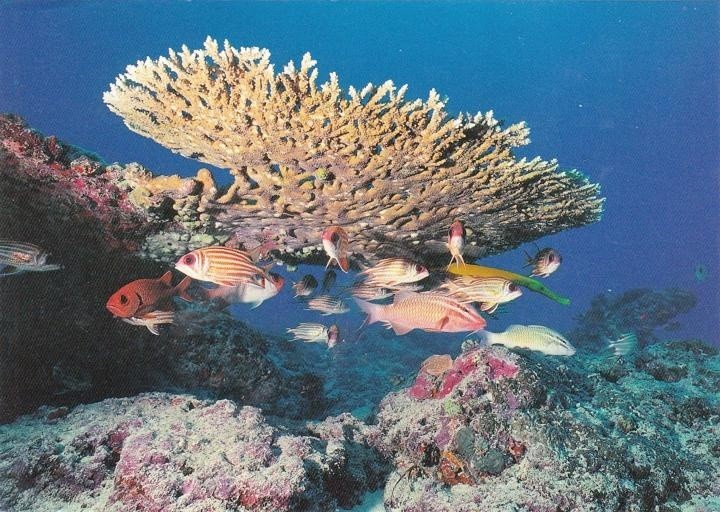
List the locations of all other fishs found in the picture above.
[106,220,523,351]
